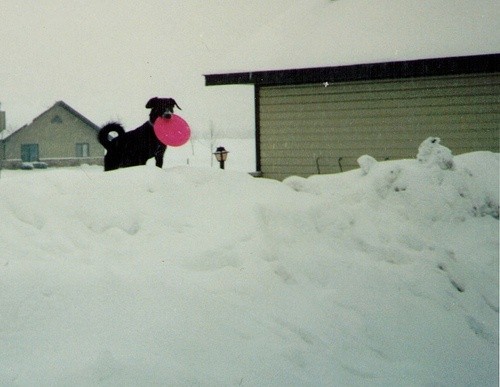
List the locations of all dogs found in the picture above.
[96,98,181,172]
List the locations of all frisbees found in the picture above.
[153,113,190,146]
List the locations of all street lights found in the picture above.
[213,145,228,167]
